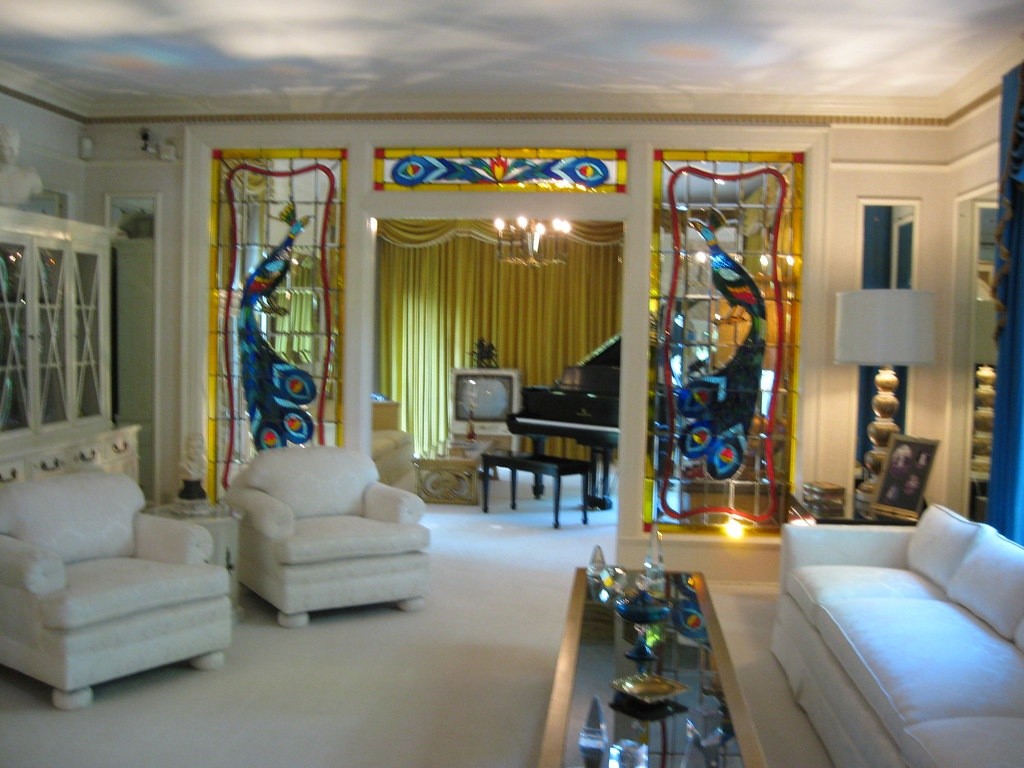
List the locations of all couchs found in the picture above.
[0,470,235,710]
[227,444,432,626]
[767,502,1024,768]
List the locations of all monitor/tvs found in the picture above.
[450,369,521,436]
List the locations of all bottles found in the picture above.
[644,525,663,590]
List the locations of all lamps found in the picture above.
[972,299,999,484]
[833,289,935,502]
[495,215,572,268]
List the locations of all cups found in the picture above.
[609,738,649,768]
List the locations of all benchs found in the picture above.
[479,449,593,529]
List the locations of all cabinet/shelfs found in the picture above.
[451,433,522,454]
[786,490,915,526]
[374,402,400,432]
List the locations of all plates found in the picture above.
[608,673,689,703]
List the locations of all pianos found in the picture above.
[505,333,619,511]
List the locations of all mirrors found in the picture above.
[954,179,1002,523]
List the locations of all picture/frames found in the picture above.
[871,433,940,520]
[103,190,160,242]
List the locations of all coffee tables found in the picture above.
[538,567,766,768]
[413,437,500,504]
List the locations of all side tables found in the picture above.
[146,505,247,618]
